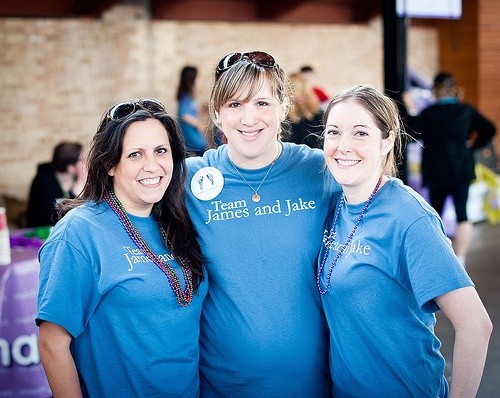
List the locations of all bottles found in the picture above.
[24,226,55,239]
[0,207,11,266]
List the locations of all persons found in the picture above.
[317,85,493,398]
[35,99,212,398]
[25,65,497,270]
[181,52,343,398]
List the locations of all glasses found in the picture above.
[215,51,279,81]
[106,98,167,123]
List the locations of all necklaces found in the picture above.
[104,191,194,307]
[229,141,279,202]
[317,176,381,295]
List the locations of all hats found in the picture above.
[431,73,454,92]
